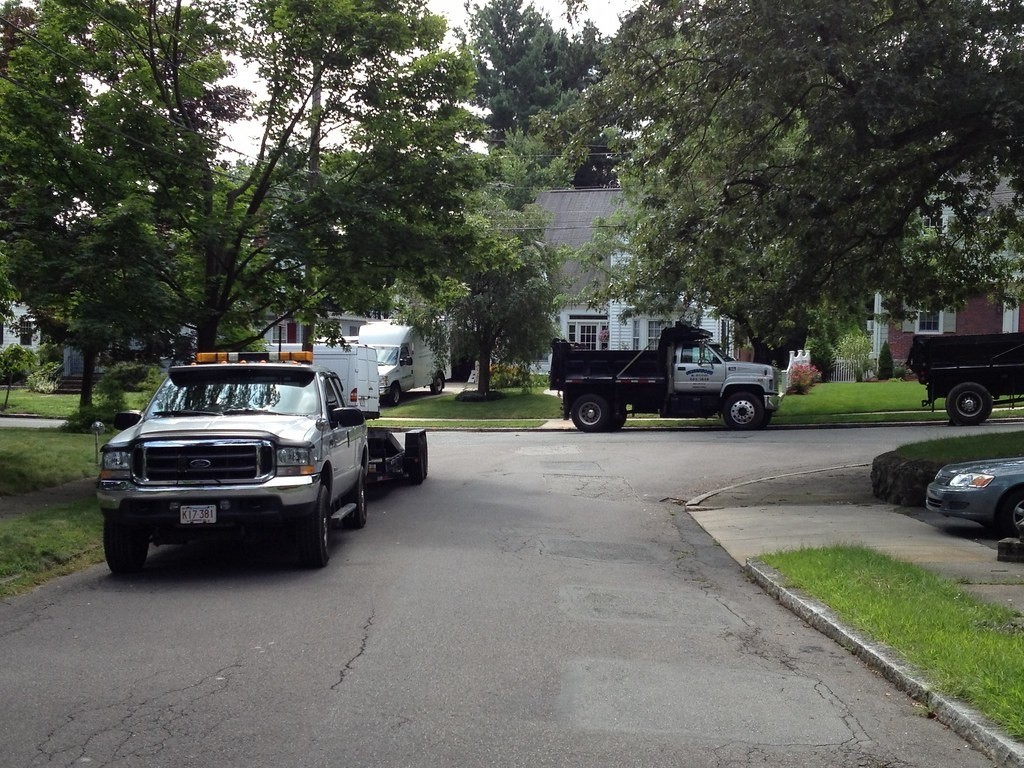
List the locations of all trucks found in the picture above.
[550,320,781,434]
[359,315,451,405]
[912,328,1024,427]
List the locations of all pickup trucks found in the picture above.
[94,350,370,574]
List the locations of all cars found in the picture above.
[924,453,1024,540]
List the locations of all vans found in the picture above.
[263,341,381,417]
